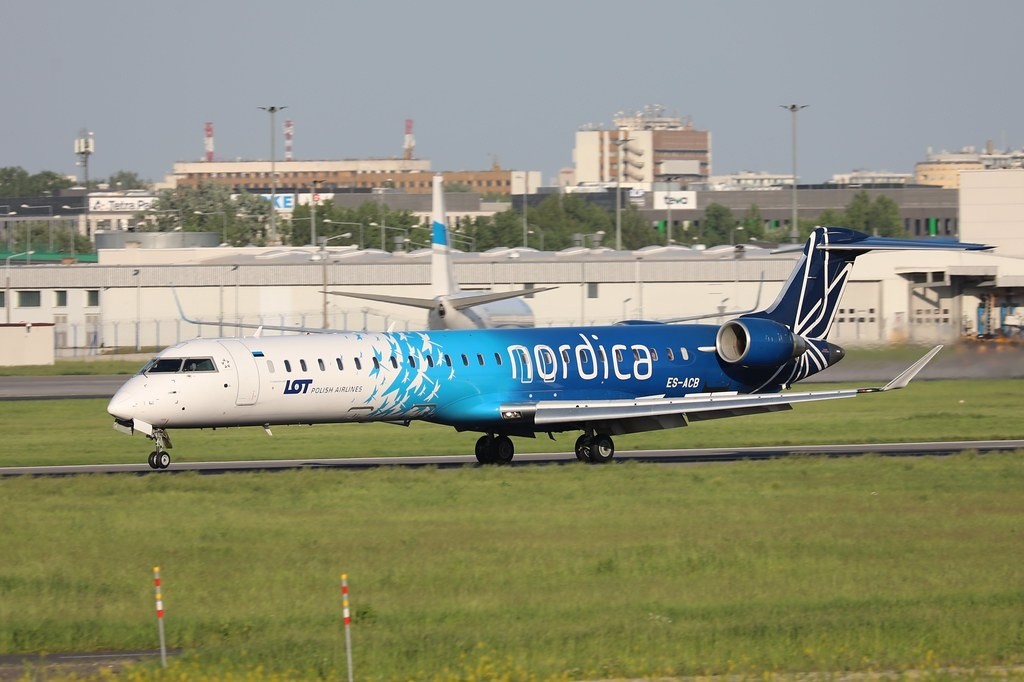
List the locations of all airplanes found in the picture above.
[106,174,999,471]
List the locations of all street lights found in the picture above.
[582,231,605,248]
[320,219,365,252]
[258,106,287,245]
[527,231,545,252]
[193,211,227,245]
[730,227,743,245]
[411,225,475,249]
[779,103,813,244]
[20,204,52,253]
[236,213,270,243]
[370,223,409,252]
[5,251,34,322]
[62,206,89,246]
